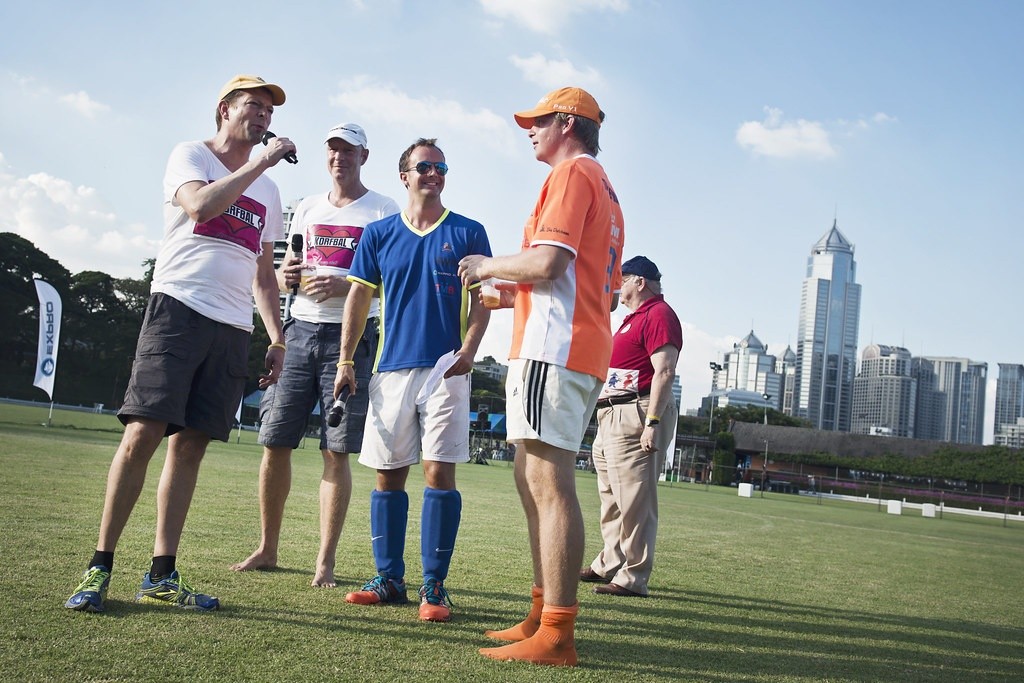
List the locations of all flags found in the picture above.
[33,279,62,402]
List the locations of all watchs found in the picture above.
[644,418,659,426]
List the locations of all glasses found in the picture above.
[405,161,449,176]
[621,277,631,287]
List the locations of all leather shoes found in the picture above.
[579,567,611,582]
[592,582,647,598]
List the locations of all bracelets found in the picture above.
[268,344,285,350]
[336,361,355,367]
[646,415,660,421]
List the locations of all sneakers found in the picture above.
[66,565,111,612]
[135,570,220,611]
[346,575,409,604]
[417,577,454,622]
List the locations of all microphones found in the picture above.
[328,384,352,428]
[291,234,303,296]
[260,131,298,164]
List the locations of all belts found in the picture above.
[596,387,650,409]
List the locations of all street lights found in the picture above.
[762,394,772,424]
[708,362,722,434]
[676,448,682,484]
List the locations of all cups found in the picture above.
[300,262,317,291]
[481,277,501,309]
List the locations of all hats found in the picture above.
[325,123,367,149]
[621,256,662,281]
[220,75,286,106]
[514,87,601,129]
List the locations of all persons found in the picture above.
[734,459,744,486]
[579,256,683,598]
[705,459,714,482]
[467,434,598,473]
[334,140,495,621]
[457,88,624,666]
[761,465,768,489]
[806,472,818,496]
[66,76,286,613]
[228,123,405,588]
